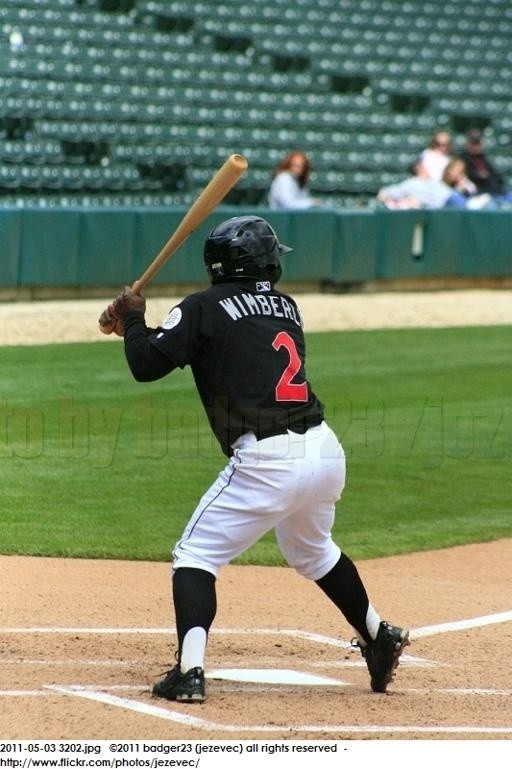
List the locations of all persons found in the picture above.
[98,215,410,706]
[266,150,329,210]
[374,124,512,209]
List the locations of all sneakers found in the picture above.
[356,618,412,693]
[151,663,207,705]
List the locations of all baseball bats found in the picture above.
[98,154,248,334]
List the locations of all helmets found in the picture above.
[204,213,294,287]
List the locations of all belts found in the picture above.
[255,415,325,441]
